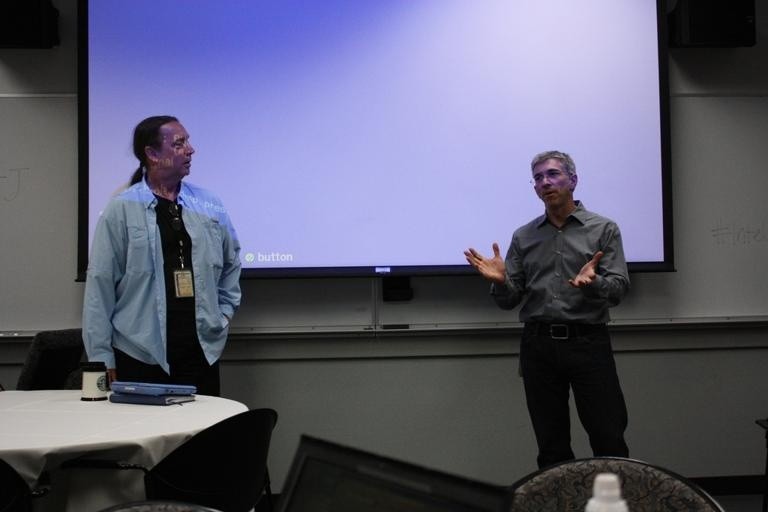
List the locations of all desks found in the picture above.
[1,386,250,492]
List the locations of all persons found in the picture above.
[82,116,243,395]
[463,151,632,470]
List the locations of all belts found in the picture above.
[525,322,606,340]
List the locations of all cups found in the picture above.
[80,361,108,402]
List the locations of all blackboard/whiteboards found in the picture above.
[1,93,767,338]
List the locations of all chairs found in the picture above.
[16,328,93,391]
[93,407,725,512]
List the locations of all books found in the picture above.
[108,382,197,406]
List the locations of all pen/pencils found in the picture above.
[382,325,409,329]
